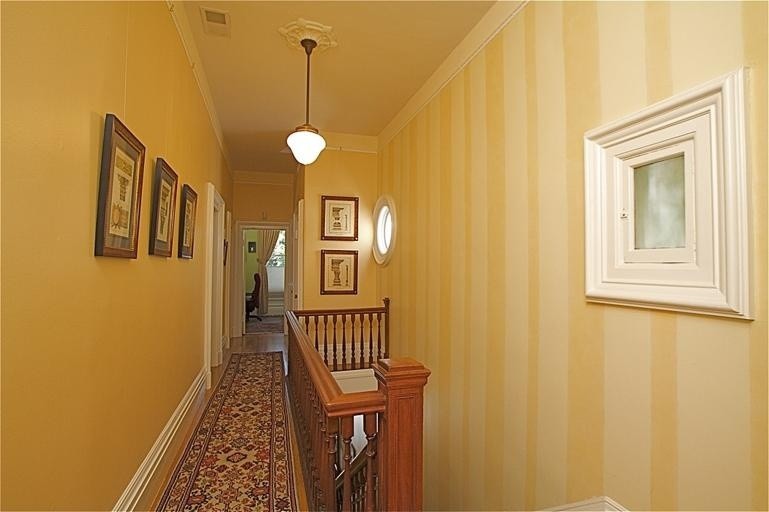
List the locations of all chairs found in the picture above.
[245,273,262,323]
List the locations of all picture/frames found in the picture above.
[93,113,146,259]
[320,195,360,241]
[178,184,197,260]
[149,158,179,258]
[320,249,358,295]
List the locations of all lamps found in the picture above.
[285,39,326,165]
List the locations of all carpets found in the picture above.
[156,350,300,512]
